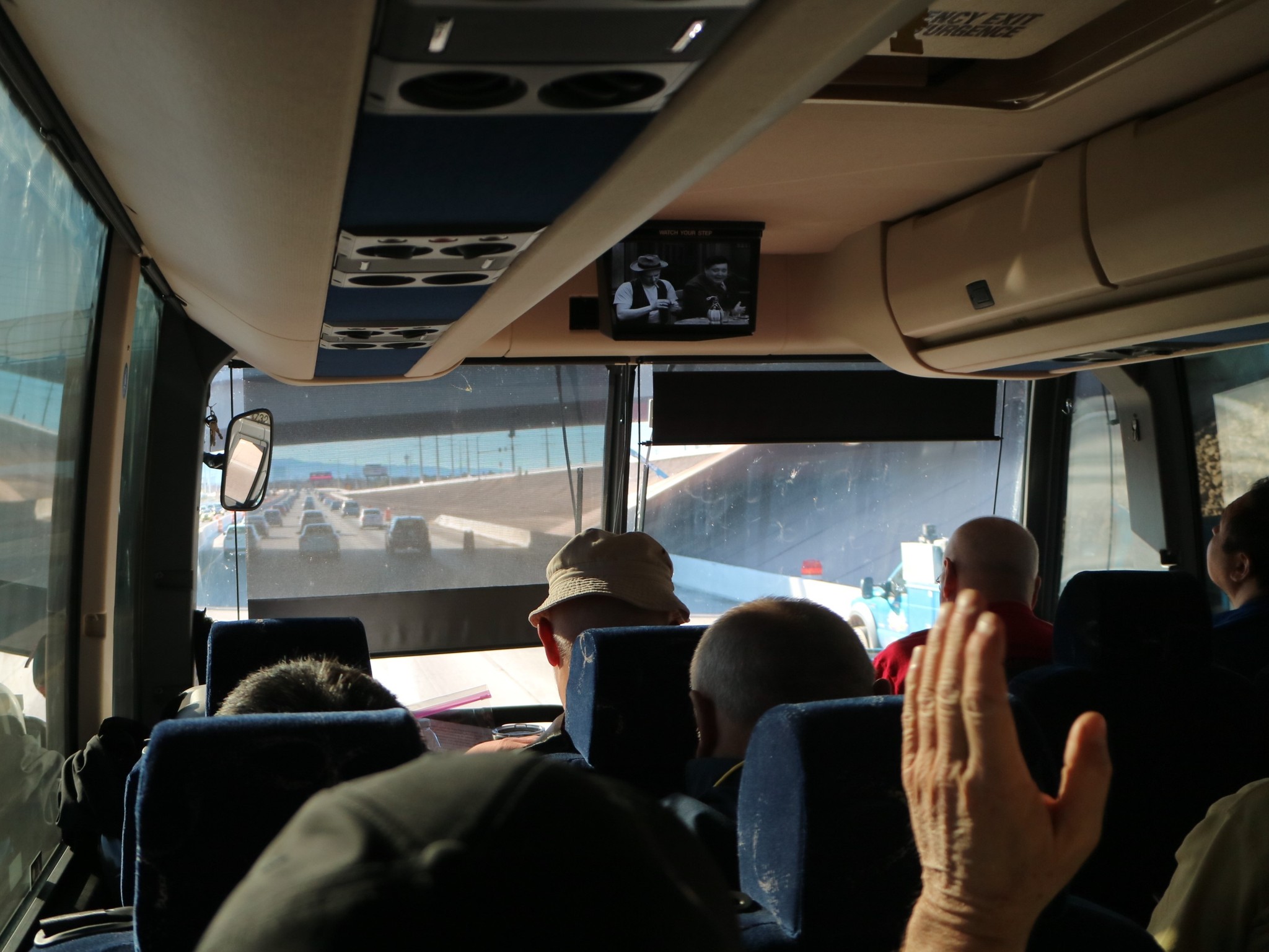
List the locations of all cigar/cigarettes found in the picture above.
[654,282,659,289]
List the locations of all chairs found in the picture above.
[32,570,1269,952]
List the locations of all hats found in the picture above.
[630,254,668,271]
[527,528,691,618]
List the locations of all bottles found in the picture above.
[418,718,441,750]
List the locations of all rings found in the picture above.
[660,303,662,306]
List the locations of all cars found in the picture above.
[264,510,283,527]
[358,508,384,529]
[198,487,332,522]
[242,515,269,539]
[296,524,340,561]
[384,516,430,555]
[339,501,361,519]
[223,524,263,560]
[330,501,341,511]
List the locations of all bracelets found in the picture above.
[729,310,733,317]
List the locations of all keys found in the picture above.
[205,415,224,447]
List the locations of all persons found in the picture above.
[616,251,749,330]
[0,718,66,877]
[61,655,424,893]
[870,478,1269,952]
[465,529,894,918]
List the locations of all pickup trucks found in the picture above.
[847,560,942,662]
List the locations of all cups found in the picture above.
[492,724,546,740]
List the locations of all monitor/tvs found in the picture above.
[597,218,765,342]
